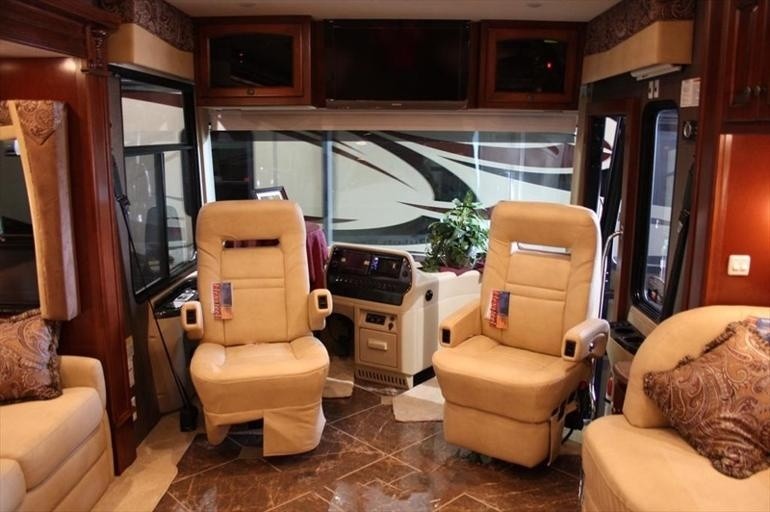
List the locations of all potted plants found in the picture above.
[416,190,492,272]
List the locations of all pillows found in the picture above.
[643,323,770,478]
[0,308,63,404]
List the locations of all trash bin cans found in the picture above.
[611,360,633,415]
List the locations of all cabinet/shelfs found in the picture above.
[193,14,311,107]
[478,18,582,108]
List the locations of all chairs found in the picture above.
[179,198,334,458]
[582,303,770,511]
[1,355,115,512]
[433,200,612,473]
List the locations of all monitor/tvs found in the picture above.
[324,19,472,110]
